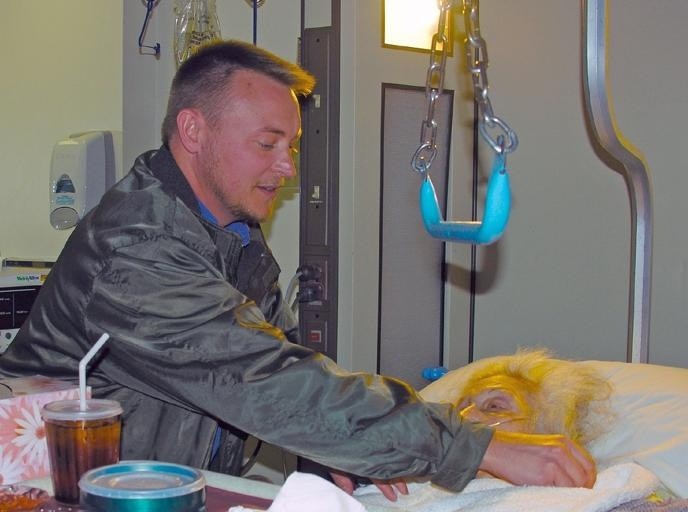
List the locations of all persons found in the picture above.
[0,38,598,501]
[451,344,613,480]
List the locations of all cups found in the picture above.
[41,397,123,504]
[75,460,207,511]
